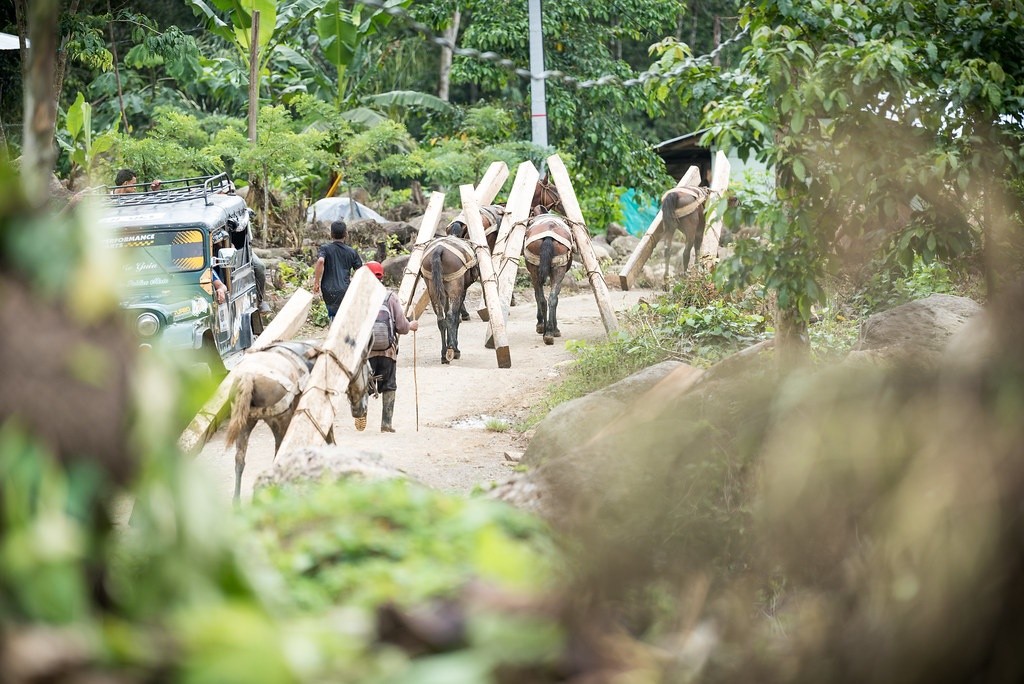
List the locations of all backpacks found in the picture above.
[368,291,396,352]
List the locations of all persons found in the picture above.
[312,221,362,321]
[110,169,161,194]
[355,261,419,435]
[179,243,228,304]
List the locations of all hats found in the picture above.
[363,261,382,278]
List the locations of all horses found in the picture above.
[446,167,566,306]
[223,332,373,509]
[421,237,479,364]
[662,187,710,281]
[524,214,572,345]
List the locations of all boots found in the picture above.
[380,391,395,433]
[356,417,365,430]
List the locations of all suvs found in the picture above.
[71,170,260,375]
